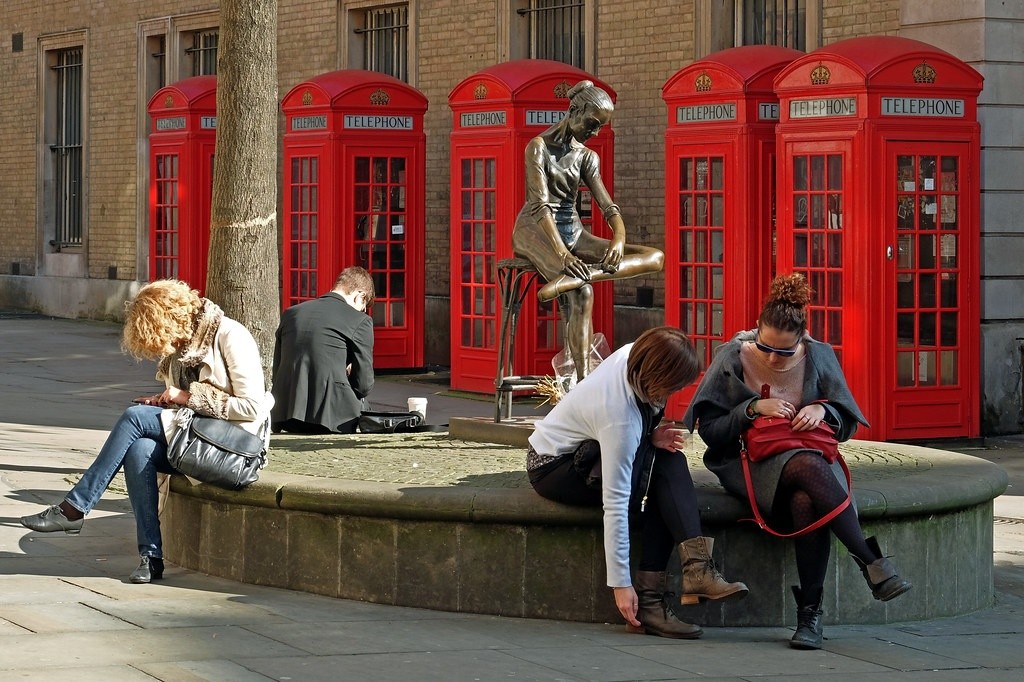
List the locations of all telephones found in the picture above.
[357,206,380,251]
[896,227,915,282]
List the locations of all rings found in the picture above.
[785,401,791,407]
[805,415,810,420]
[780,410,783,414]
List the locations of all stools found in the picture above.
[490,256,573,424]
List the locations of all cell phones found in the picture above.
[132,397,167,405]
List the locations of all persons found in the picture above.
[682,273,912,647]
[269,267,375,433]
[527,326,750,640]
[511,80,666,384]
[20,278,272,583]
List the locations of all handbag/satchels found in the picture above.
[166,413,267,490]
[744,399,838,465]
[359,407,449,433]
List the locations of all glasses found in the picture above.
[755,329,802,357]
[358,290,372,308]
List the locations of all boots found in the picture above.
[789,585,824,649]
[848,536,913,602]
[677,535,749,606]
[626,570,704,638]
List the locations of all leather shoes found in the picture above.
[130,556,164,583]
[19,504,85,535]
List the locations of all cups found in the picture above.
[407,397,428,420]
